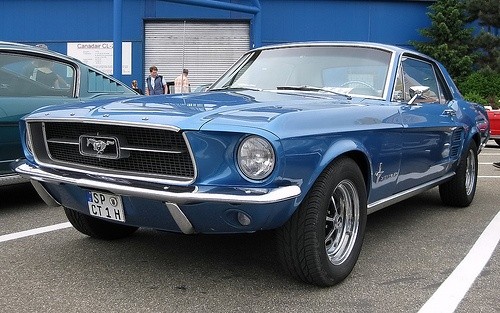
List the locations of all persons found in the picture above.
[131,79,145,96]
[174,69,190,94]
[144,66,168,96]
[29,59,60,89]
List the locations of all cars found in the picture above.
[9,40,491,289]
[0,41,142,192]
